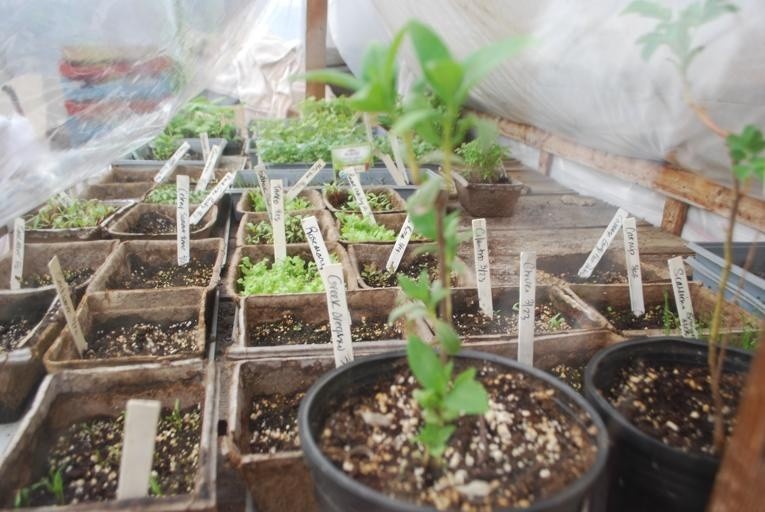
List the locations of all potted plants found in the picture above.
[0,20,765,512]
